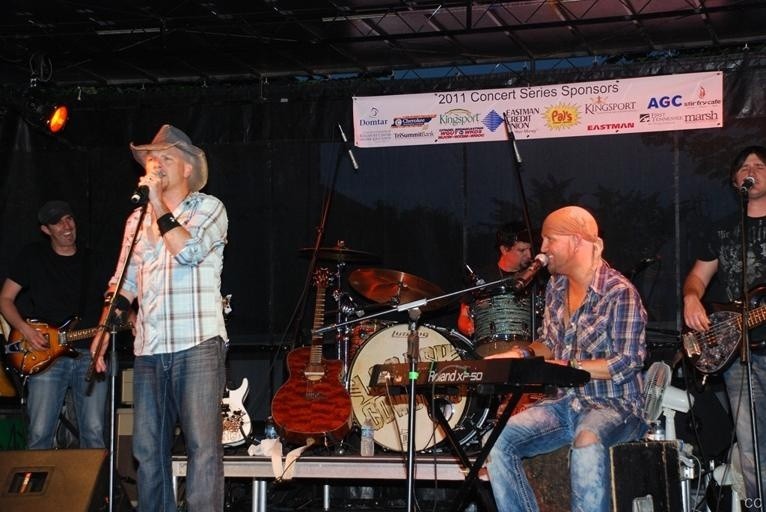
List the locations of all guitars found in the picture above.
[678,306,766,375]
[221,293,255,446]
[0,290,233,399]
[270,261,352,447]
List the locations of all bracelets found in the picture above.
[516,347,532,357]
[565,358,579,368]
[104,292,130,313]
[156,213,178,233]
[95,324,106,329]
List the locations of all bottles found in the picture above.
[654,421,665,442]
[264,415,275,439]
[644,421,654,441]
[361,418,374,456]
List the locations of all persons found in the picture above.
[0,200,118,473]
[682,145,765,512]
[455,221,542,335]
[487,206,651,512]
[89,124,230,512]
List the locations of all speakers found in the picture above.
[1,447,134,511]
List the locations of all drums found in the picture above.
[344,322,489,452]
[473,289,534,351]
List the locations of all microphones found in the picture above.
[333,119,361,173]
[741,175,754,192]
[512,253,550,291]
[501,117,525,173]
[127,170,162,204]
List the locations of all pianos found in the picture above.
[368,355,592,398]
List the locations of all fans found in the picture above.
[640,361,695,480]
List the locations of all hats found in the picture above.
[38,200,76,225]
[130,124,209,192]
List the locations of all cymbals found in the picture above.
[349,267,447,308]
[305,241,365,257]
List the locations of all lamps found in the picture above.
[16,92,67,134]
